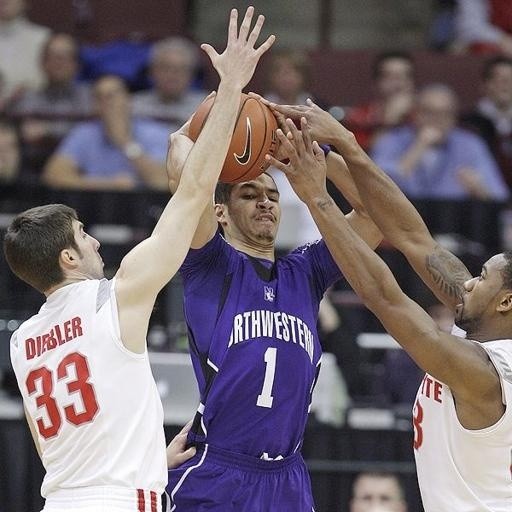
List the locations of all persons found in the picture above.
[368,1,511,200]
[168,90,383,512]
[265,98,512,512]
[1,30,333,192]
[350,469,408,512]
[4,6,275,512]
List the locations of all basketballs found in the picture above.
[188,94,278,184]
